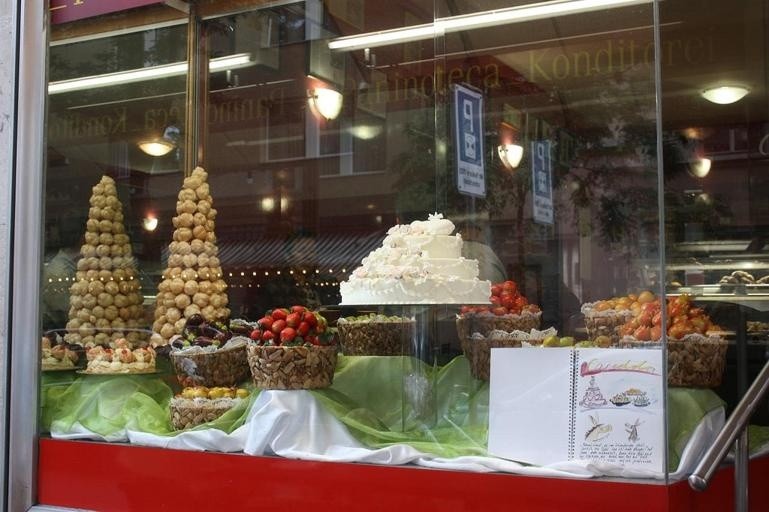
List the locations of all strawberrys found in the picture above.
[251,306,336,347]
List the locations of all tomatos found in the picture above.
[460,280,539,316]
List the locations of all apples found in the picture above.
[595,290,655,311]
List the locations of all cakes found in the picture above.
[338,212,493,305]
[43,170,231,373]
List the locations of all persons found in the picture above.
[511,254,586,336]
[437,218,510,351]
[252,228,346,323]
[42,208,90,330]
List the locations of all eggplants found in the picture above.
[174,313,254,347]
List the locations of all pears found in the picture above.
[543,334,612,347]
[182,385,248,400]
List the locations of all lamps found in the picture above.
[326,0,655,57]
[306,81,345,123]
[702,79,750,106]
[137,140,175,158]
[47,52,256,95]
[497,142,525,168]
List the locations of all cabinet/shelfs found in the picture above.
[627,257,768,425]
